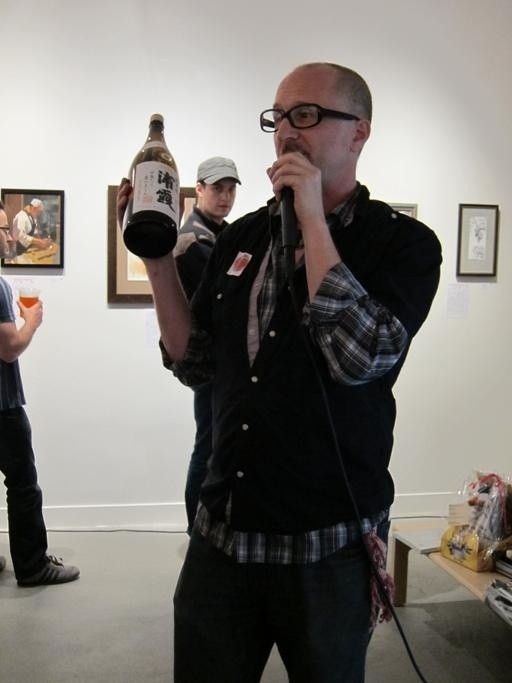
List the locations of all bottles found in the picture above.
[123,113,181,259]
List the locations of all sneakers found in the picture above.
[16,556,79,587]
[0,557,6,572]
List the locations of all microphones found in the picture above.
[278,184,298,249]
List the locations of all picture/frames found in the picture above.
[383,202,418,222]
[0,188,65,268]
[456,203,499,277]
[106,184,197,304]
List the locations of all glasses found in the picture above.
[260,103,362,132]
[0,224,9,234]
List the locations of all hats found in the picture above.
[30,198,42,207]
[197,157,242,186]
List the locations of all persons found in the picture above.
[114,62,444,683]
[11,197,54,257]
[171,156,243,536]
[0,204,81,588]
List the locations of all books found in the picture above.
[391,526,448,555]
[492,550,512,580]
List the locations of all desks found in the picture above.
[384,514,512,632]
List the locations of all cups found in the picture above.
[17,282,41,320]
